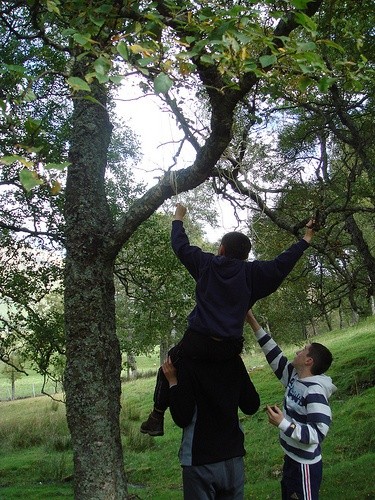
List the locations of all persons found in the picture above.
[161,352,261,500]
[246,309,338,500]
[140,202,316,437]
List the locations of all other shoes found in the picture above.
[140,414,164,436]
